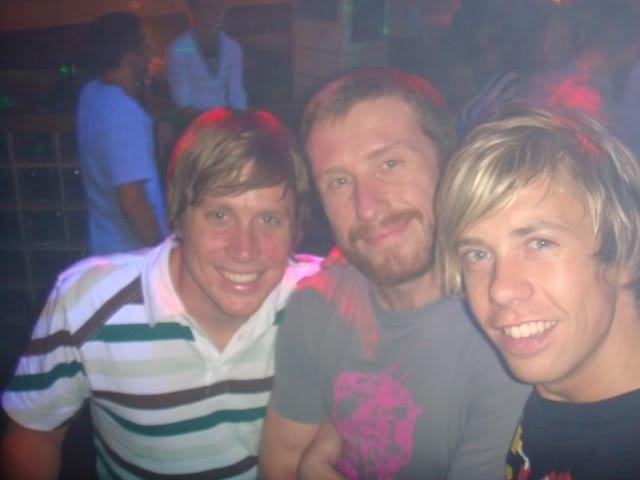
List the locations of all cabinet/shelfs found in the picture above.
[0,111,88,328]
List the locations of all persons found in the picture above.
[1,107,346,480]
[78,2,639,265]
[433,91,638,480]
[257,66,534,479]
